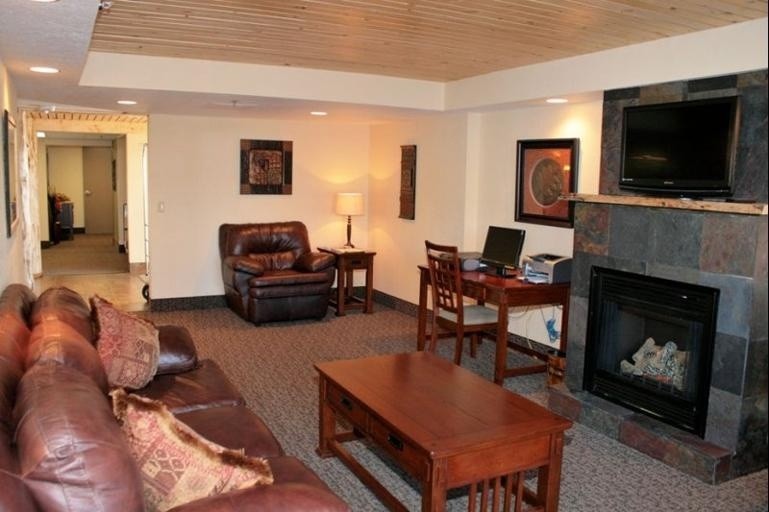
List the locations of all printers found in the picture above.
[517,253,572,285]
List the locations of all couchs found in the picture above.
[0,281,354,512]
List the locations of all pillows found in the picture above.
[109,381,275,512]
[85,293,160,389]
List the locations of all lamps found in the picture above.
[334,192,366,250]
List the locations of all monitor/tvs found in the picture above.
[480,225,526,278]
[618,93,742,198]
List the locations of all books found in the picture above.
[334,247,365,254]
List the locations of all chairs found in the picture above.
[217,220,335,328]
[423,239,509,365]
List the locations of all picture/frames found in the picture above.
[4,111,22,237]
[239,137,294,197]
[513,137,582,230]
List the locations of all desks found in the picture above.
[316,245,377,316]
[416,263,572,388]
